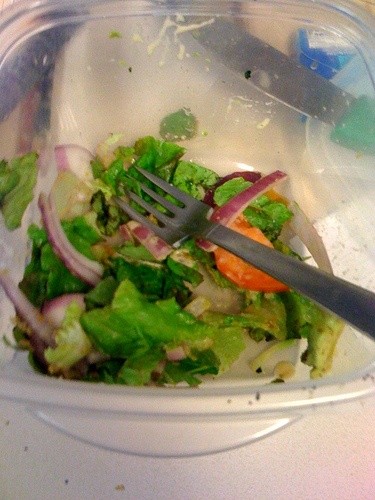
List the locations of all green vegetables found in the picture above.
[0,136,343,389]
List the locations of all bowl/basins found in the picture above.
[0,0,375,461]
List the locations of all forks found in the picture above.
[112,164,374,343]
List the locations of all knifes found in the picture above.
[184,14,375,155]
